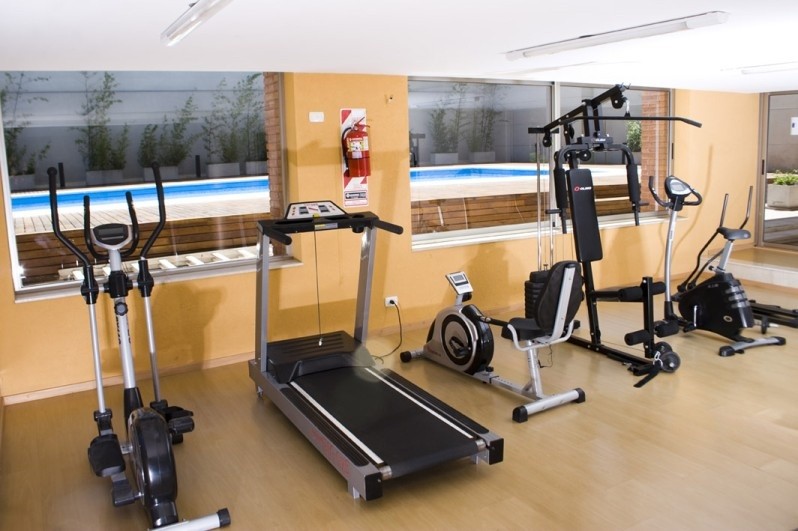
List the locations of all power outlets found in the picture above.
[384,296,399,307]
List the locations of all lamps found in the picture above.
[162,0,238,46]
[502,10,729,61]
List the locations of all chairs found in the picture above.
[568,168,642,348]
[501,260,583,396]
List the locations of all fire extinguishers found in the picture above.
[341,117,371,178]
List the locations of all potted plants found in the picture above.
[69,71,130,186]
[623,120,643,164]
[137,95,208,182]
[766,169,798,210]
[203,73,251,179]
[243,94,270,175]
[464,84,511,162]
[0,70,55,191]
[428,82,466,165]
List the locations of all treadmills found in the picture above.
[249,199,503,500]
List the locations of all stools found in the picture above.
[714,225,751,274]
